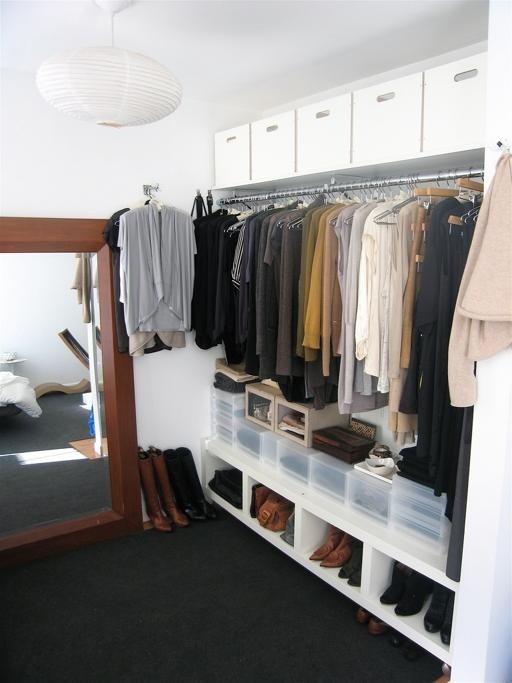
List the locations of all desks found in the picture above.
[1,358,29,378]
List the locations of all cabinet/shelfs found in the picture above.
[419,47,487,175]
[248,106,298,186]
[211,119,250,189]
[349,70,424,181]
[292,89,354,186]
[197,436,463,668]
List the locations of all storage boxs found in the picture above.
[212,371,449,559]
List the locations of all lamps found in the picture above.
[33,0,187,128]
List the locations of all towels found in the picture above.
[72,251,93,325]
[446,150,511,409]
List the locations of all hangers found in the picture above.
[209,164,486,277]
[110,182,166,226]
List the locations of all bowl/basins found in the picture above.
[365,456,394,474]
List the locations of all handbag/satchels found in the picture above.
[256,492,293,532]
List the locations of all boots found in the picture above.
[136,445,223,534]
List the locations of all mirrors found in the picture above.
[1,212,144,577]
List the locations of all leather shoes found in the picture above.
[309,523,362,588]
[380,560,452,645]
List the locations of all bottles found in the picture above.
[87,404,96,436]
